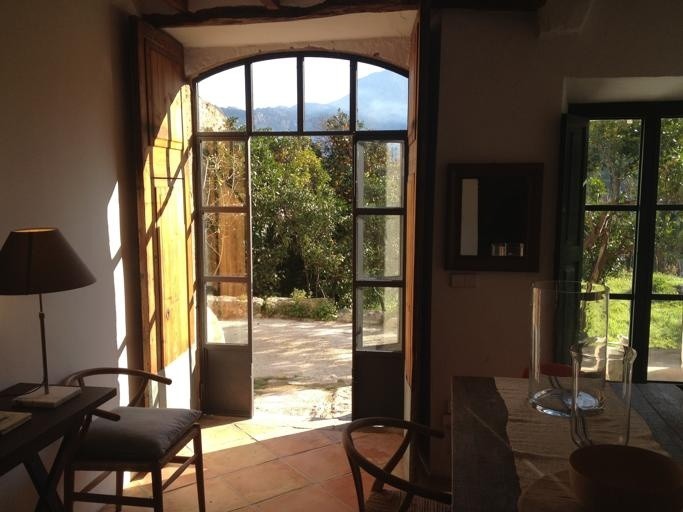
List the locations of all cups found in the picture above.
[568,341,638,448]
[527,279,610,419]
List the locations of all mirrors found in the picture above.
[443,160,543,275]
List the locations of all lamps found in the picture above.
[0,227,96,409]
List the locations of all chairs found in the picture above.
[58,367,205,512]
[344,416,452,512]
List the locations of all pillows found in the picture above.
[70,408,203,463]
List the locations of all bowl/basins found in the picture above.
[568,444,683,512]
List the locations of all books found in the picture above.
[0,410,32,436]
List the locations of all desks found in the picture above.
[451,376,683,512]
[0,382,116,512]
